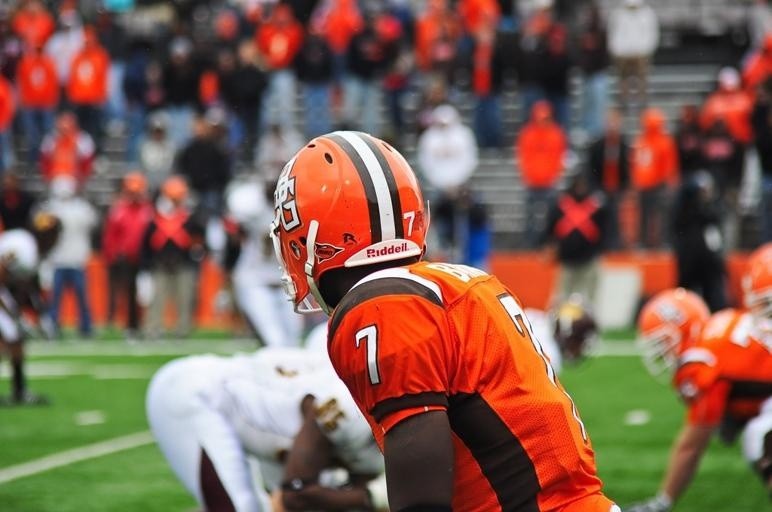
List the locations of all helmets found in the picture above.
[637,288,711,357]
[272,131,425,313]
[739,242,771,310]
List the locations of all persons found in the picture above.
[1,213,63,404]
[272,132,620,512]
[1,2,772,340]
[145,176,390,512]
[635,210,772,512]
